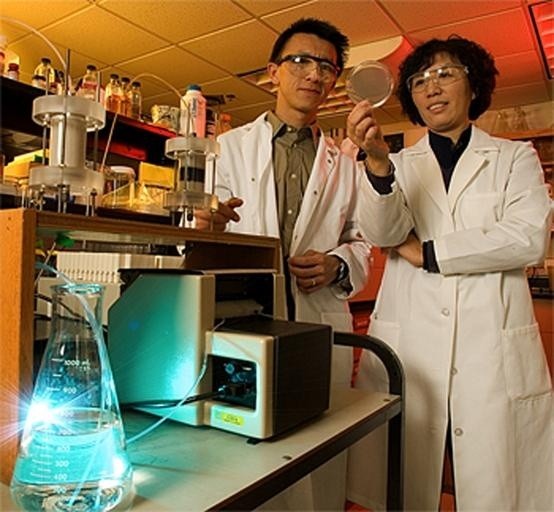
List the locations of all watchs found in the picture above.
[337,261,349,282]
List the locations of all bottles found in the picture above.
[120,76,130,101]
[6,63,20,81]
[78,64,98,103]
[104,72,122,115]
[0,52,6,76]
[125,81,143,120]
[179,84,207,138]
[31,57,56,93]
[9,281,137,512]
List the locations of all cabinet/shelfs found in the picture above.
[1,75,406,512]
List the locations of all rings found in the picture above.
[312,279,317,287]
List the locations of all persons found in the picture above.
[179,15,373,386]
[344,33,554,512]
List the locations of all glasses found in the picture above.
[406,63,469,93]
[276,54,342,82]
